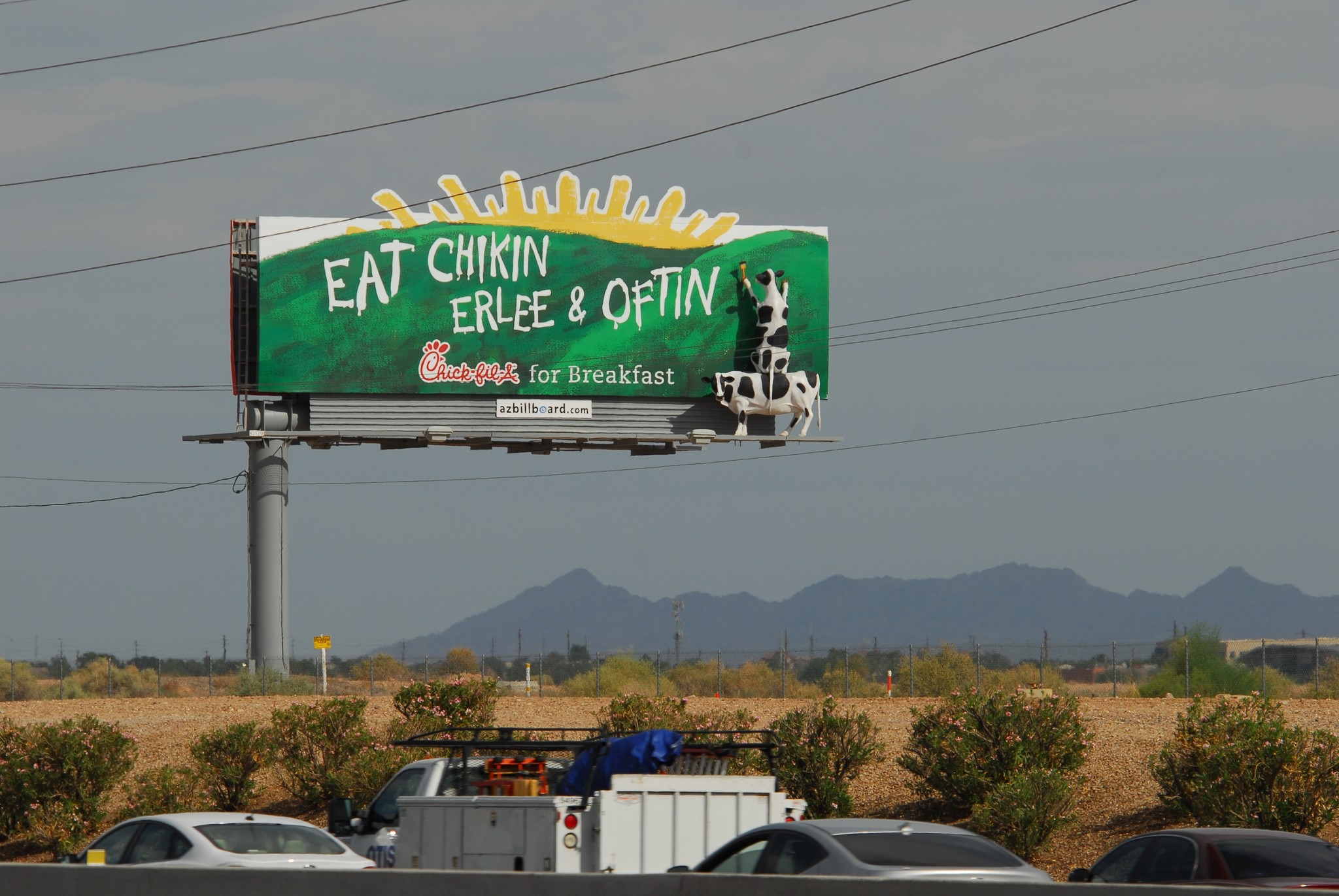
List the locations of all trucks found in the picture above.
[321,727,808,880]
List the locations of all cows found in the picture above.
[742,267,791,415]
[701,369,822,438]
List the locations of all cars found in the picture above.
[1067,827,1338,896]
[56,810,380,871]
[665,818,1051,883]
